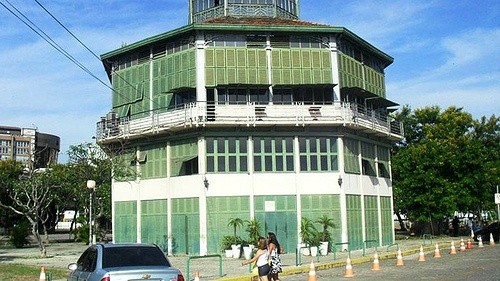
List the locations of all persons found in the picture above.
[266,232,282,281]
[242,237,270,281]
[452,216,472,237]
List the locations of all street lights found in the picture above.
[87,180,97,247]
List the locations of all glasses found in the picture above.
[267,237,270,239]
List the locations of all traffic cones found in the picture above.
[343,255,355,278]
[307,260,318,281]
[372,249,380,270]
[395,248,404,265]
[434,233,495,256]
[418,243,427,261]
[38,267,47,281]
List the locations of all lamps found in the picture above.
[204,176,209,188]
[338,174,342,186]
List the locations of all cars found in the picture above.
[65,242,185,281]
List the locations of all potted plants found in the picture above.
[222,218,262,260]
[301,213,335,256]
